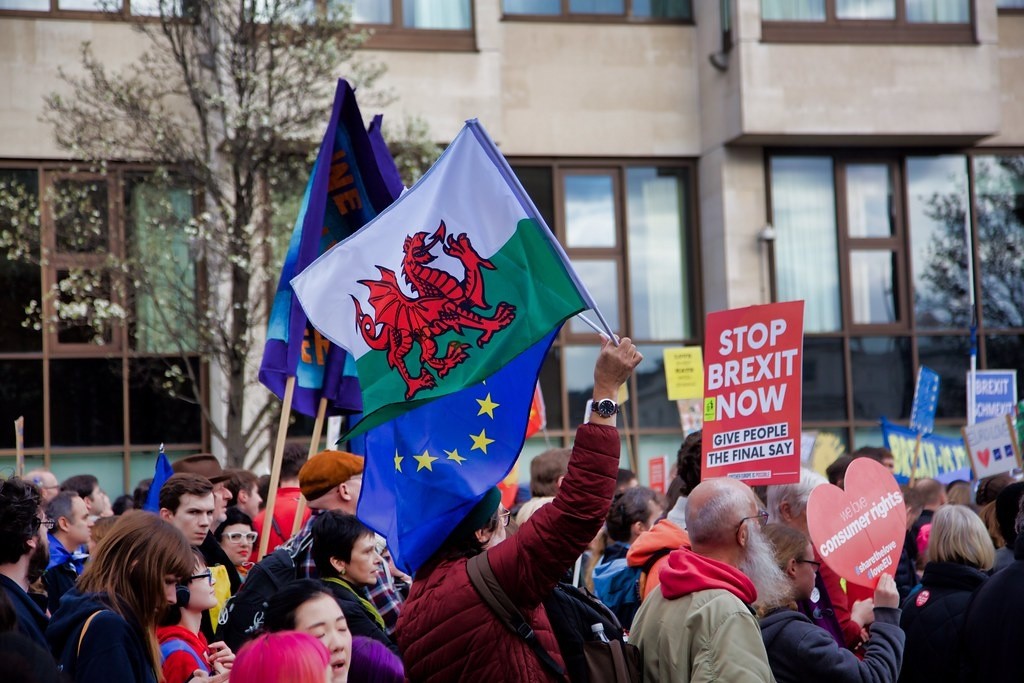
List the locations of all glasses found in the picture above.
[797,559,821,572]
[221,532,258,543]
[43,485,60,493]
[736,511,769,534]
[38,518,55,529]
[494,510,511,526]
[189,568,212,586]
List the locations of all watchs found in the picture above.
[591,398,620,417]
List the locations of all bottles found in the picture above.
[591,623,610,643]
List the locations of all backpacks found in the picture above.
[216,532,314,655]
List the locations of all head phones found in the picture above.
[175,583,190,606]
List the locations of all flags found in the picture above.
[147,80,595,578]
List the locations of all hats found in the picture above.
[171,453,243,479]
[298,449,364,501]
[450,485,501,532]
[916,523,932,555]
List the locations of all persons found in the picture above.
[0,336,1024,683]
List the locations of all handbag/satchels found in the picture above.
[465,551,644,683]
